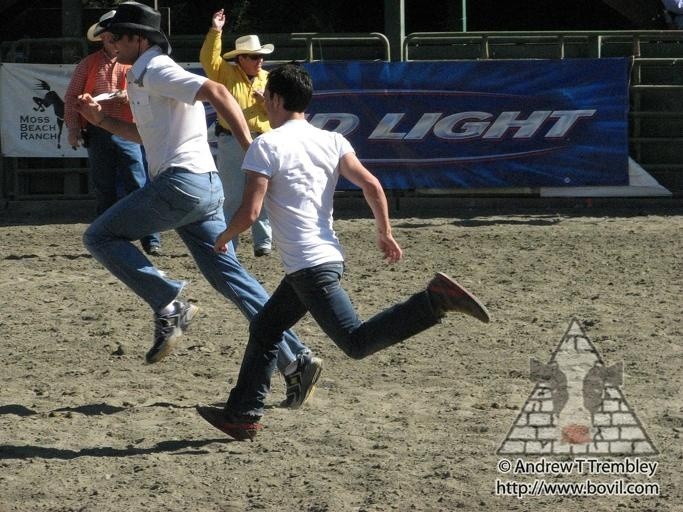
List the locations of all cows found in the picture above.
[529,350,624,445]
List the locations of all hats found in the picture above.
[223,35,275,59]
[88,2,173,55]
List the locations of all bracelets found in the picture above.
[95,116,107,129]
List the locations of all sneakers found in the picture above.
[196,403,259,440]
[427,272,490,324]
[255,248,272,256]
[146,300,199,364]
[280,353,324,409]
[147,244,161,256]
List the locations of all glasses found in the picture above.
[246,54,264,60]
[111,33,124,41]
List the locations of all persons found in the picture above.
[63,11,162,255]
[71,0,324,410]
[199,8,274,257]
[195,62,490,441]
